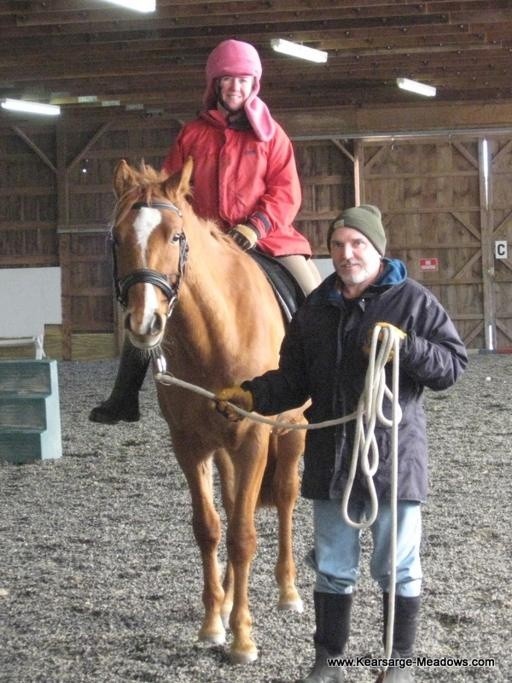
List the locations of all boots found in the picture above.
[88,332,153,426]
[295,589,348,683]
[373,591,421,683]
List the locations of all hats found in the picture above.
[206,39,263,80]
[326,203,388,260]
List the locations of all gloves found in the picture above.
[362,321,408,365]
[227,210,273,253]
[210,386,254,423]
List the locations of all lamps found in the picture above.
[271,37,328,66]
[0,97,61,118]
[394,77,438,98]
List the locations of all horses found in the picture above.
[105,156,324,666]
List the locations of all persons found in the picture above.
[86,37,321,426]
[208,200,472,681]
